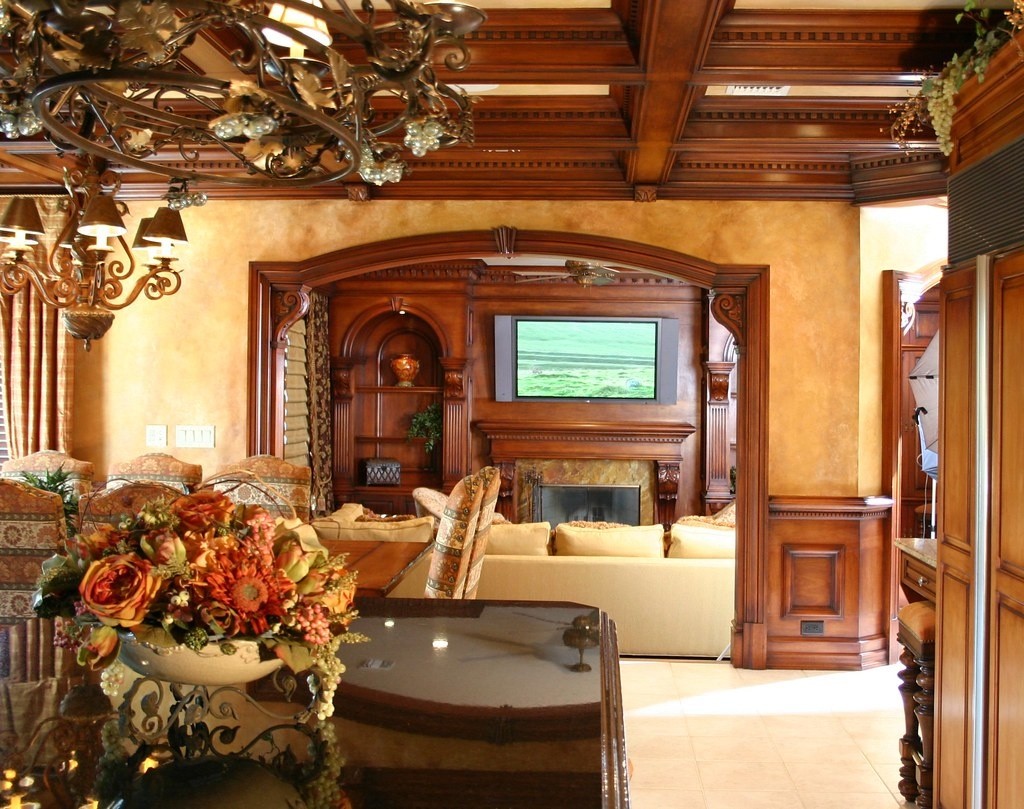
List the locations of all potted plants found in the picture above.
[408,400,443,478]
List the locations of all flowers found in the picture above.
[20,456,511,684]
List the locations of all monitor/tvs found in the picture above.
[494,315,678,406]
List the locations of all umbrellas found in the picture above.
[906,327,939,483]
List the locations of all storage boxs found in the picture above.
[364,458,403,488]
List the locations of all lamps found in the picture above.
[0,0,501,353]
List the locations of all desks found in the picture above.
[891,535,937,612]
[318,540,435,600]
[0,596,632,809]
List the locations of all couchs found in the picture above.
[311,498,738,659]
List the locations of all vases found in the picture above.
[391,353,422,388]
[97,616,296,684]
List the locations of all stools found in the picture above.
[896,599,936,809]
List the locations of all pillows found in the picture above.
[662,499,737,554]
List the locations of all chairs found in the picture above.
[0,448,313,623]
